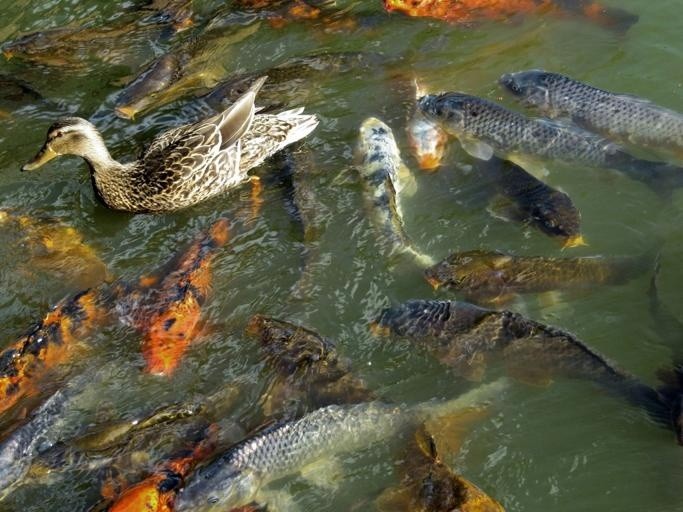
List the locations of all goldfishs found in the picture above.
[0,0,681,512]
